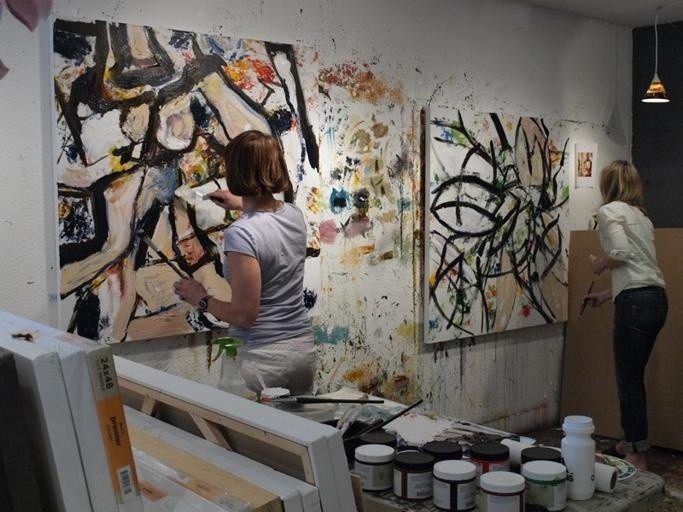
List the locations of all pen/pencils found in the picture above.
[354,398,425,436]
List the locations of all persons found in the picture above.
[173,130,315,401]
[581,160,668,470]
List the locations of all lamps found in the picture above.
[640,3,670,105]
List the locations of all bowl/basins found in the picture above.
[319,419,371,462]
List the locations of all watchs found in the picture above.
[197,295,212,312]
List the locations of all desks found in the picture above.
[305,388,664,510]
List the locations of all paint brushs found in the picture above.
[270,397,386,404]
[578,279,595,320]
[143,237,190,281]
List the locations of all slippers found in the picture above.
[601,446,627,458]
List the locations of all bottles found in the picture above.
[355,415,596,512]
